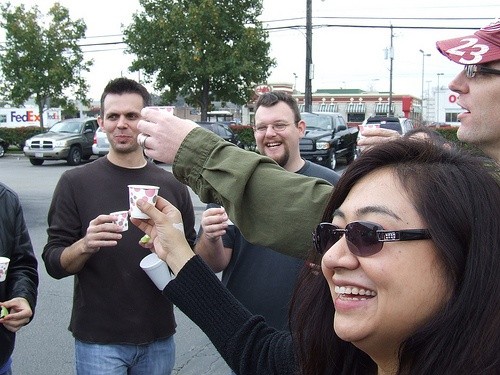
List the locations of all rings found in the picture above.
[139,135,149,150]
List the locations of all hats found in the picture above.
[436,21,500,65]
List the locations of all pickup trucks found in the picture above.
[297,111,358,171]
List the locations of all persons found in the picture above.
[41,78,196,375]
[129,18,500,375]
[0,183,38,375]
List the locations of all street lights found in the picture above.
[419,49,431,99]
[437,73,444,122]
[293,72,298,85]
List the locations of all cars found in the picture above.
[0,138,8,158]
[24,118,99,166]
[427,121,450,127]
[354,115,413,160]
[195,121,245,151]
[91,127,110,158]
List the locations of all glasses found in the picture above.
[311,222,432,257]
[253,121,295,132]
[464,64,500,77]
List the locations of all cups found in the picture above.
[127,184,161,220]
[221,206,234,226]
[139,249,172,290]
[144,106,175,115]
[110,210,128,232]
[358,123,381,151]
[0,256,11,281]
